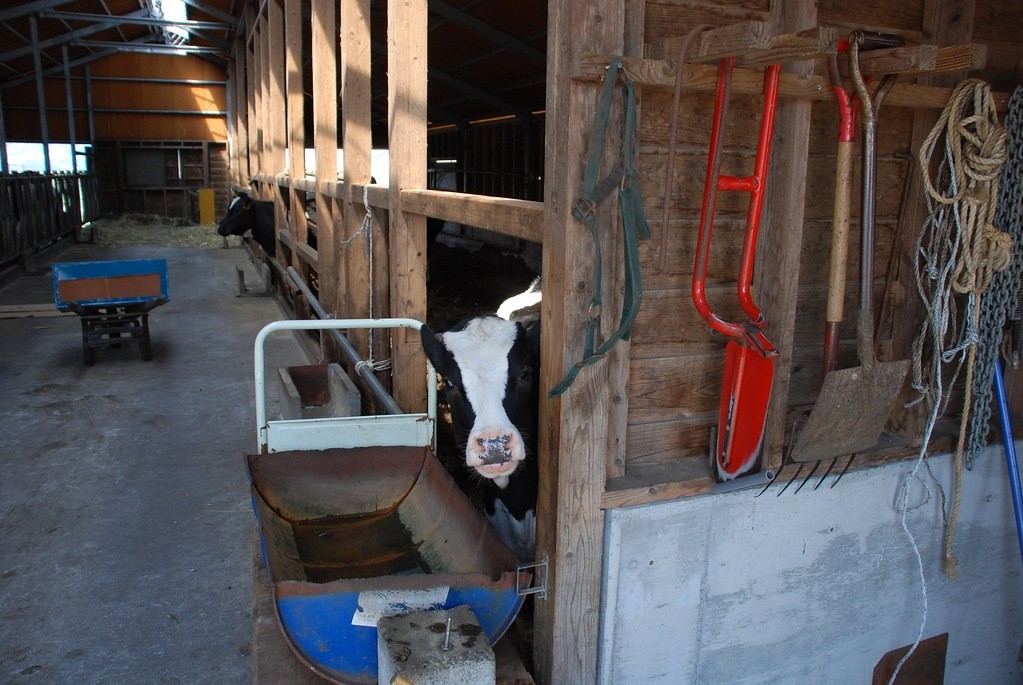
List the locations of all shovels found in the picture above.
[790,28,909,465]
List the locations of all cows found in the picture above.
[215,186,318,276]
[419,241,542,564]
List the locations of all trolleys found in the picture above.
[51,258,171,368]
[243,317,534,683]
